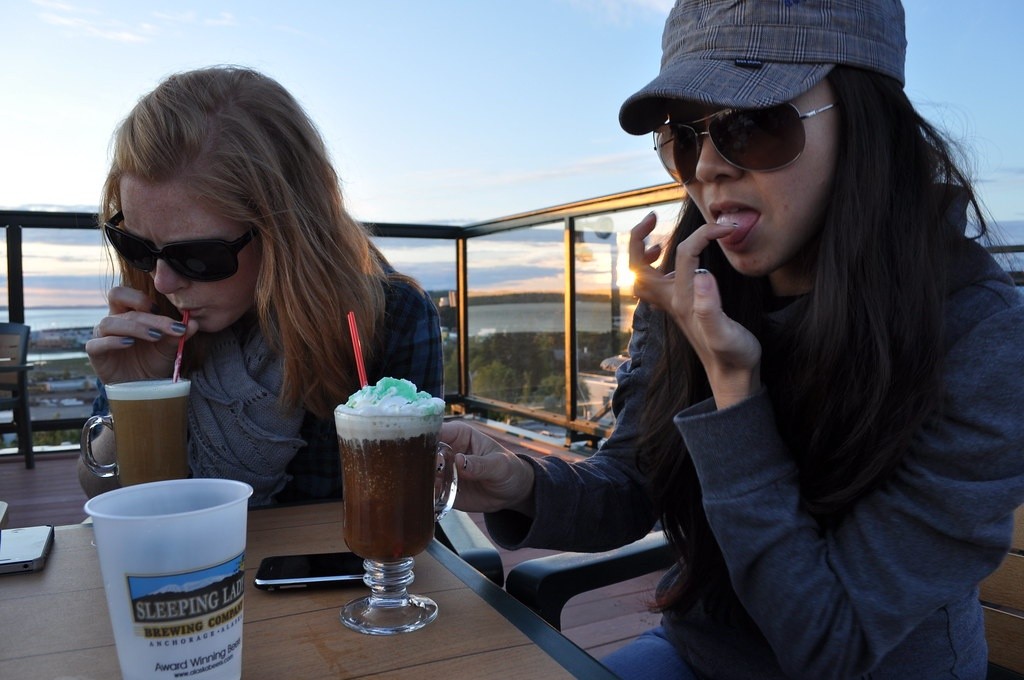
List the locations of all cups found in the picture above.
[83,478,254,680]
[334,409,458,637]
[79,376,191,487]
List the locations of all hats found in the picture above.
[618,0,906,135]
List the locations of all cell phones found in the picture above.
[254,552,369,593]
[0,523,54,575]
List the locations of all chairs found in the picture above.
[1,322,37,471]
[434,502,1024,680]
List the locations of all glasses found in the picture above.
[103,209,259,283]
[652,103,840,185]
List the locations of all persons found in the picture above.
[75,62,447,511]
[432,0,1024,680]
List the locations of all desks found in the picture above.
[0,498,621,680]
